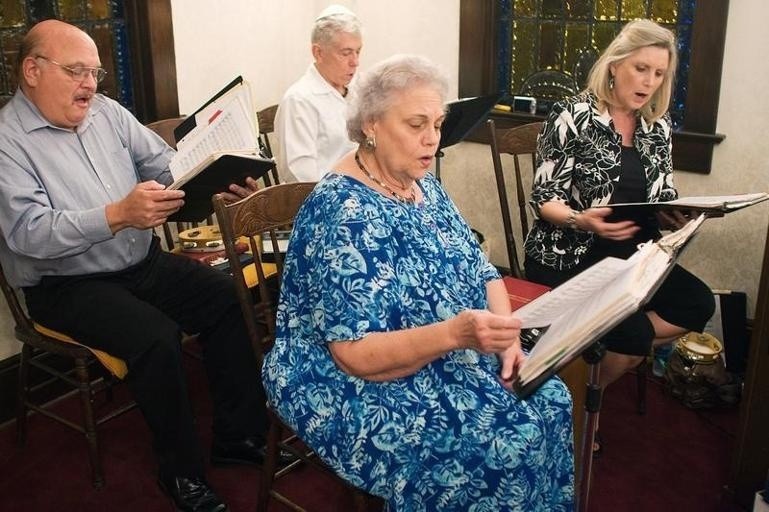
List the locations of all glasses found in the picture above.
[31,53,107,83]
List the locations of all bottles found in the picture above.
[652,342,673,378]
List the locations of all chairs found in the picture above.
[488,115,649,393]
[213,182,421,511]
[1,104,286,498]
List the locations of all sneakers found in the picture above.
[210,433,269,456]
[158,467,225,511]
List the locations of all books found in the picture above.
[588,188,768,219]
[154,72,279,223]
[511,211,715,403]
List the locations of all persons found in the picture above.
[0,18,308,511]
[257,53,580,511]
[515,16,715,455]
[269,4,382,193]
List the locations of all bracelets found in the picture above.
[564,210,583,227]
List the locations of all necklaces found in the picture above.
[351,152,418,205]
